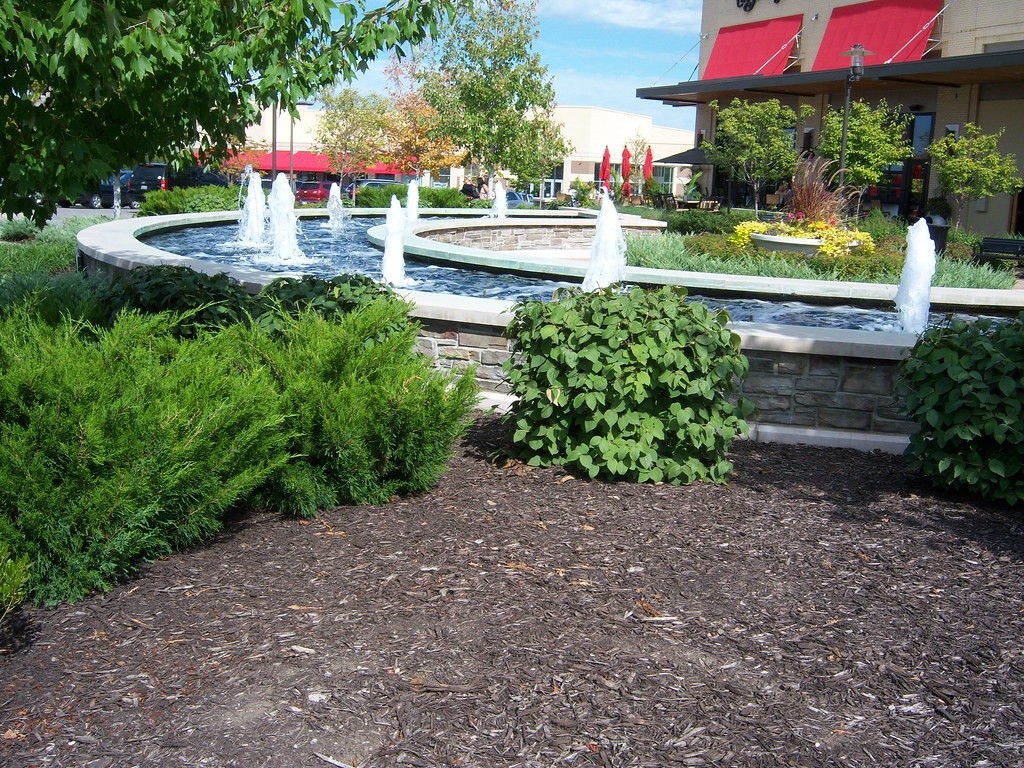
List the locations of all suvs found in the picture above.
[344,178,410,206]
[127,161,235,210]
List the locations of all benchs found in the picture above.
[973,236,1024,267]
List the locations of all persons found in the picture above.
[775,181,794,212]
[472,178,489,200]
[461,177,477,198]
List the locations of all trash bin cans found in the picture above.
[924,216,951,260]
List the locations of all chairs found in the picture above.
[870,199,890,216]
[651,193,690,212]
[767,194,780,210]
[698,194,722,212]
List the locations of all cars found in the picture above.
[505,190,537,211]
[295,182,350,204]
[56,168,134,210]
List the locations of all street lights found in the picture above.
[290,100,314,193]
[835,43,873,201]
